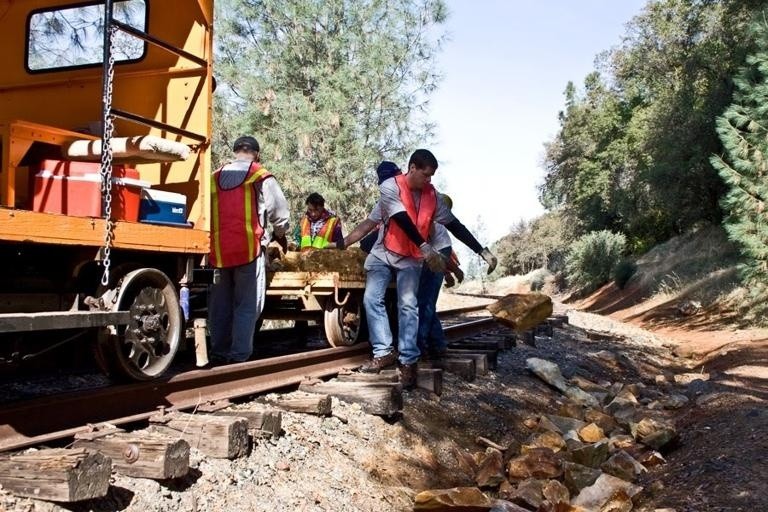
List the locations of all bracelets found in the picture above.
[335,237,347,250]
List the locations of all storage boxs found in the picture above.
[31,160,143,224]
[139,185,189,225]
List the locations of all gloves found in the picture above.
[321,239,347,250]
[421,244,450,272]
[480,247,498,274]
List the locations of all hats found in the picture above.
[233,137,260,152]
[377,161,400,184]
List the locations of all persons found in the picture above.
[281,193,344,350]
[360,149,497,388]
[208,135,290,367]
[321,160,454,361]
[438,193,464,289]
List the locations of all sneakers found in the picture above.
[398,362,418,388]
[358,351,397,372]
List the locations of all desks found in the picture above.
[0,119,102,211]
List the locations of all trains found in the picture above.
[0,0,216,388]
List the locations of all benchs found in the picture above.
[67,134,192,163]
